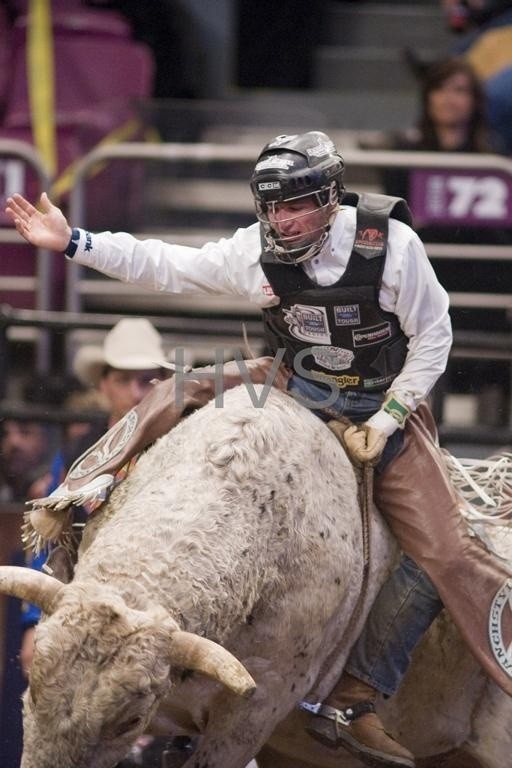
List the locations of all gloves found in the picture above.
[343,390,412,469]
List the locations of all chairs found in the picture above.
[2,12,152,316]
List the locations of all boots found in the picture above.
[305,670,415,768]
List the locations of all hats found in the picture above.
[72,318,166,388]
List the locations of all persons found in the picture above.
[353,53,512,429]
[4,127,486,766]
[11,313,190,686]
[0,371,72,501]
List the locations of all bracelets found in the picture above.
[62,228,79,260]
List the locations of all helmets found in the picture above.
[249,130,346,212]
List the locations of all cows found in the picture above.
[0,382,512,768]
